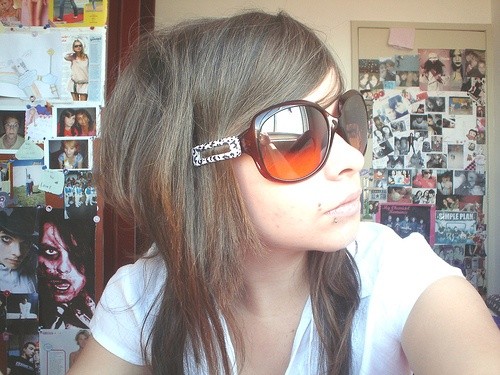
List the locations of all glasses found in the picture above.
[75,45,82,47]
[191,89,369,184]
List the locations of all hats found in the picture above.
[0,208,39,237]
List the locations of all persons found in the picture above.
[25,173,34,197]
[64,39,89,101]
[64,172,98,209]
[0,207,39,294]
[57,109,96,136]
[15,341,38,375]
[19,296,36,319]
[58,140,84,169]
[0,115,25,149]
[69,330,90,371]
[0,0,21,27]
[65,11,500,375]
[359,49,488,302]
[40,209,96,330]
[56,0,79,21]
